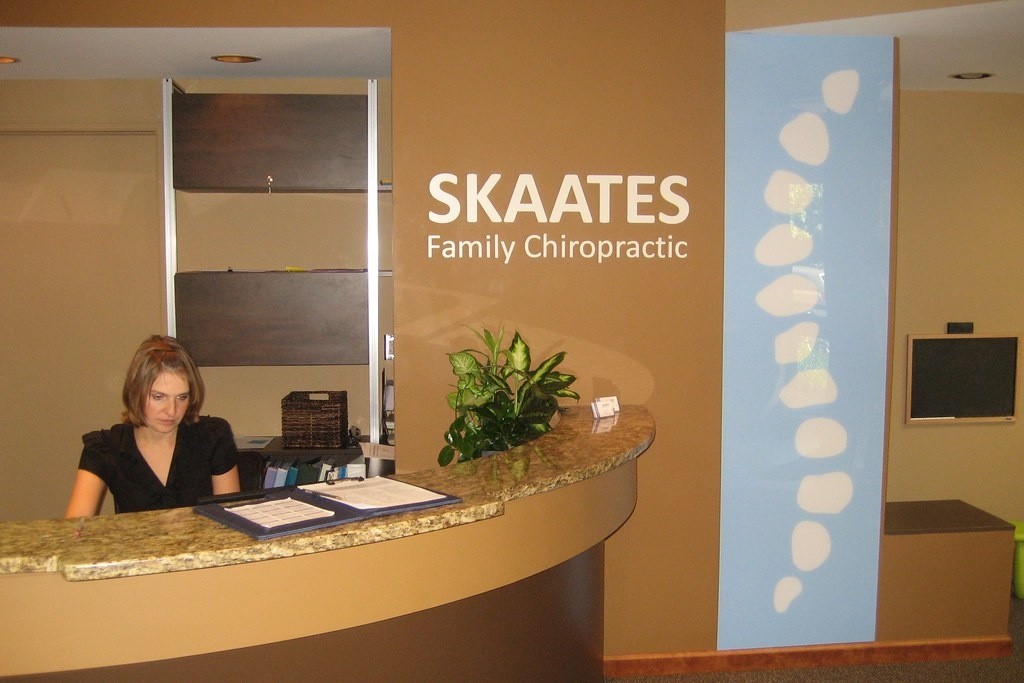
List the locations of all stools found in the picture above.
[1006,520,1024,599]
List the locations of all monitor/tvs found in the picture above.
[904,334,1020,423]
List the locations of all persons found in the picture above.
[64,334,241,520]
